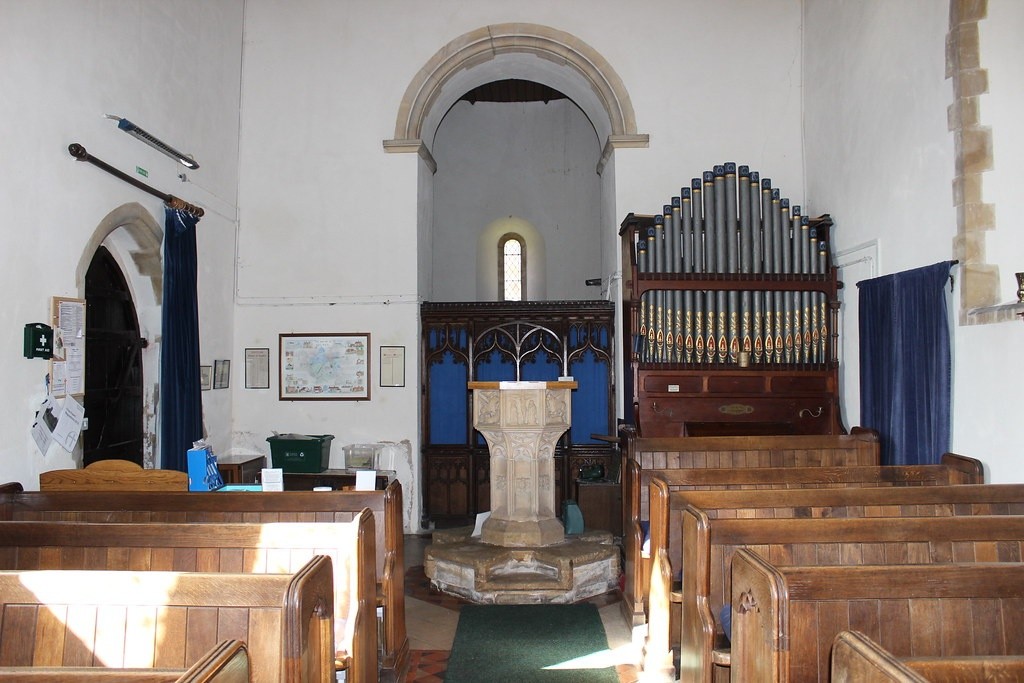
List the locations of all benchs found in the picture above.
[0,459,410,683]
[621,424,1023,683]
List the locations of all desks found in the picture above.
[216,456,265,484]
[256,470,397,490]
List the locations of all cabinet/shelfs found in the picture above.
[618,211,848,439]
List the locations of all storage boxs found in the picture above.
[342,444,385,474]
[265,434,335,473]
